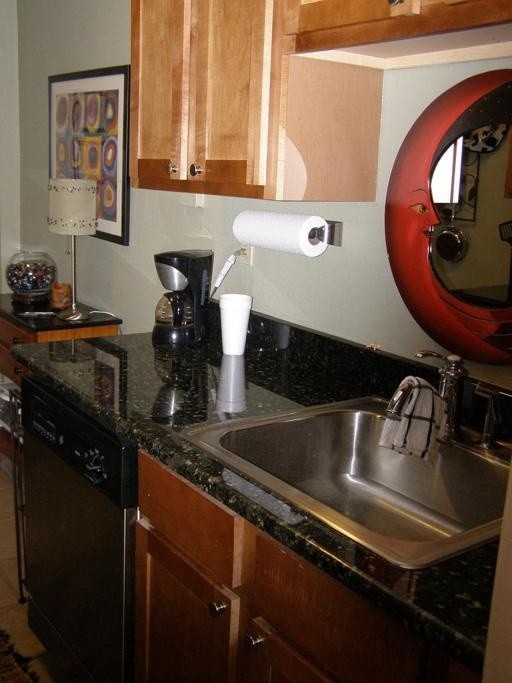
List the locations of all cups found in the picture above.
[218,293,253,355]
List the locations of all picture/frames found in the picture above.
[46,61,132,247]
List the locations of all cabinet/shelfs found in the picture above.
[293,0,512,59]
[126,0,379,209]
[0,292,124,445]
[132,448,478,682]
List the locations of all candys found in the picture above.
[6,259,55,290]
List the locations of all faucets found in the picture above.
[387,349,470,438]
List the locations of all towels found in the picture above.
[377,375,449,470]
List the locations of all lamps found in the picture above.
[45,177,98,324]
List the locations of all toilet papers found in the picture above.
[232,210,330,260]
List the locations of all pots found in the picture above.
[435,203,469,264]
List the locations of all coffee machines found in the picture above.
[150,249,214,351]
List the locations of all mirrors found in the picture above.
[381,67,512,367]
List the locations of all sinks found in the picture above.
[183,402,511,562]
